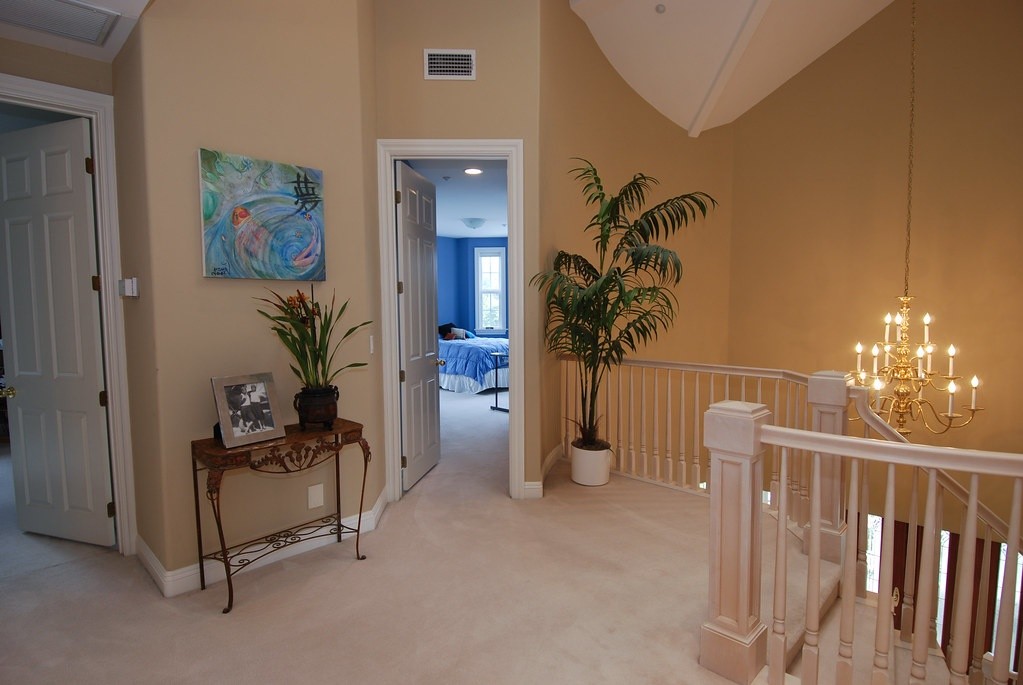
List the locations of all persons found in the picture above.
[238,384,267,434]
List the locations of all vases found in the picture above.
[293,385,339,431]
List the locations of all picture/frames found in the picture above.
[211,372,287,448]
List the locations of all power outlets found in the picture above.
[308,483,324,509]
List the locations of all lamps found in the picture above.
[462,219,486,229]
[848,1,985,437]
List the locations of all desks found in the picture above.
[191,418,371,613]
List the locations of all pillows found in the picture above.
[438,323,476,340]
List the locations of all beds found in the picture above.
[438,323,509,394]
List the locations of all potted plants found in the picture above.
[528,157,720,487]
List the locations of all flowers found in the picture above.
[252,283,373,391]
